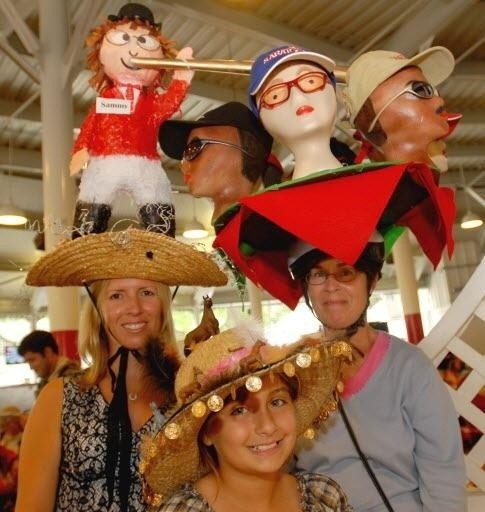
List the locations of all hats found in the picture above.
[25,230,228,286]
[345,47,455,128]
[139,326,353,498]
[247,45,336,116]
[159,101,272,160]
[213,162,456,311]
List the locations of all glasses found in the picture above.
[257,71,336,115]
[183,138,255,159]
[306,262,360,283]
[368,81,441,131]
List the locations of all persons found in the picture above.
[68,3,195,243]
[351,112,464,177]
[143,372,352,512]
[5,326,83,442]
[277,253,469,512]
[247,45,346,182]
[160,101,280,225]
[15,277,186,512]
[342,43,456,163]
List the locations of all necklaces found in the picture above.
[126,386,144,400]
